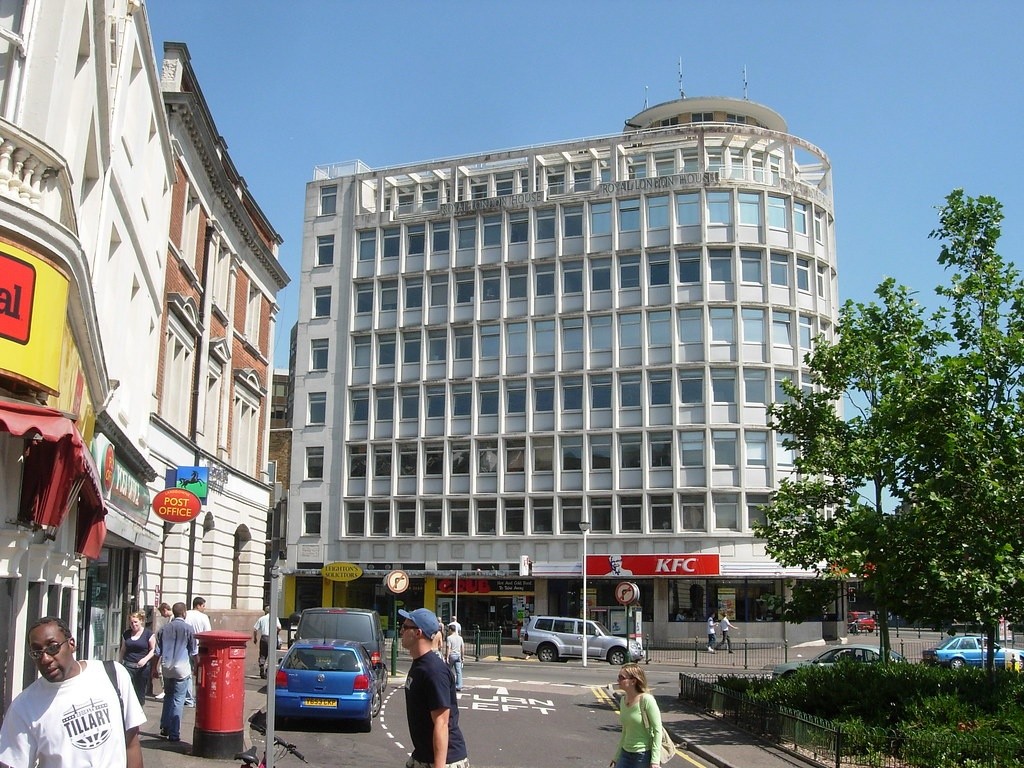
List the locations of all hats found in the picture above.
[398,608,438,640]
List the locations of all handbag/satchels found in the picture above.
[648,723,676,765]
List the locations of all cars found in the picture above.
[848,612,875,633]
[772,645,903,679]
[922,636,1024,670]
[275,638,386,733]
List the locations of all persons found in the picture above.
[676,609,698,621]
[431,616,461,650]
[398,608,470,768]
[446,625,464,691]
[254,606,281,678]
[150,602,199,741]
[0,616,147,768]
[706,611,738,653]
[610,662,662,768]
[119,597,212,707]
[852,621,859,635]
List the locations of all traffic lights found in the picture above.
[849,587,855,602]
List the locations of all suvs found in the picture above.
[519,615,643,666]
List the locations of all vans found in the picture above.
[291,608,388,696]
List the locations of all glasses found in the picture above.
[400,622,419,630]
[618,675,631,681]
[28,639,68,660]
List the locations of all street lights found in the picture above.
[579,521,590,667]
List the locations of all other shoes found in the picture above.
[728,650,735,654]
[159,729,181,744]
[260,673,268,678]
[706,645,717,654]
[155,692,166,700]
[188,703,196,707]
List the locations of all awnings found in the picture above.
[0,400,109,561]
